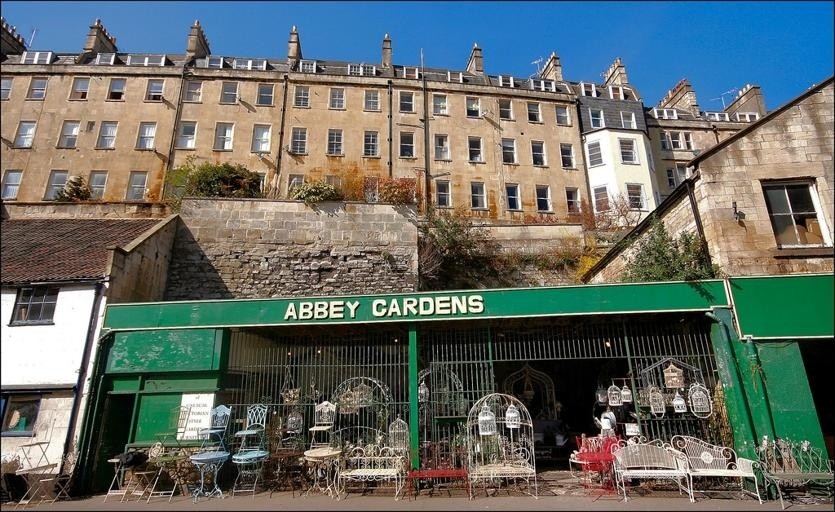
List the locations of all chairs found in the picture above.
[16,404,766,507]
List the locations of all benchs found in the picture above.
[753,434,835,511]
